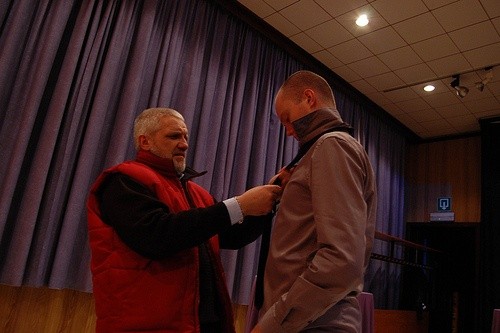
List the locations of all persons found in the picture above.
[248,70,377,333]
[85,107,291,333]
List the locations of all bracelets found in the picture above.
[233,195,245,225]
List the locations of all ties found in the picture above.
[252,124,353,313]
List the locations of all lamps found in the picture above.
[449,75,469,98]
[473,66,495,93]
